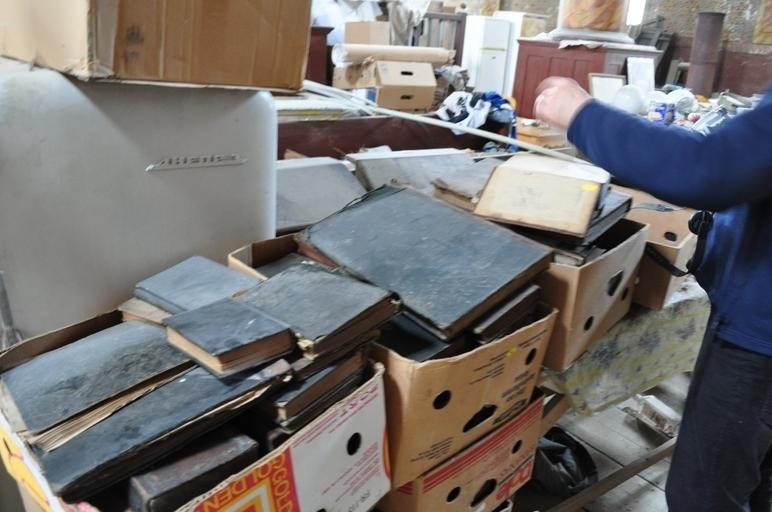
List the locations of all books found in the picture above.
[0,149,633,512]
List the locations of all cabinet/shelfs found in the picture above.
[511,40,664,119]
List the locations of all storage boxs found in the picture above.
[432,163,701,374]
[345,21,390,46]
[373,61,438,113]
[1,0,312,95]
[229,232,560,510]
[332,62,374,90]
[1,253,394,511]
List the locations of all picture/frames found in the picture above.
[587,72,626,103]
[751,0,772,45]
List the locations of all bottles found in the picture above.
[633,393,682,438]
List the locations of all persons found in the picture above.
[311,0,385,48]
[533,76,771,511]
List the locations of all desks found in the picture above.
[306,26,335,85]
